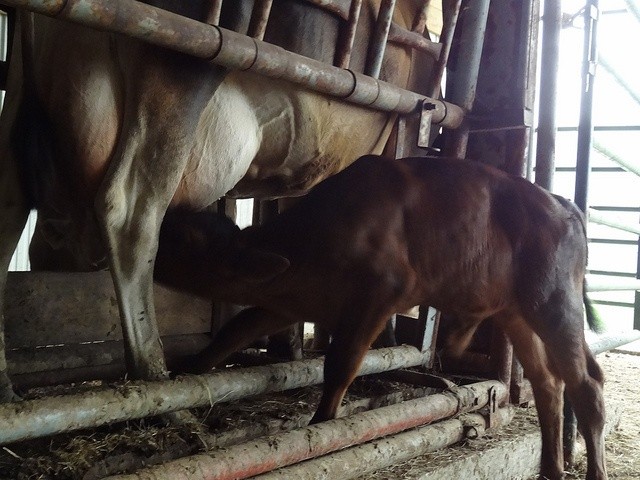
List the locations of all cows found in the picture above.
[1,0,425,427]
[152,154,607,480]
